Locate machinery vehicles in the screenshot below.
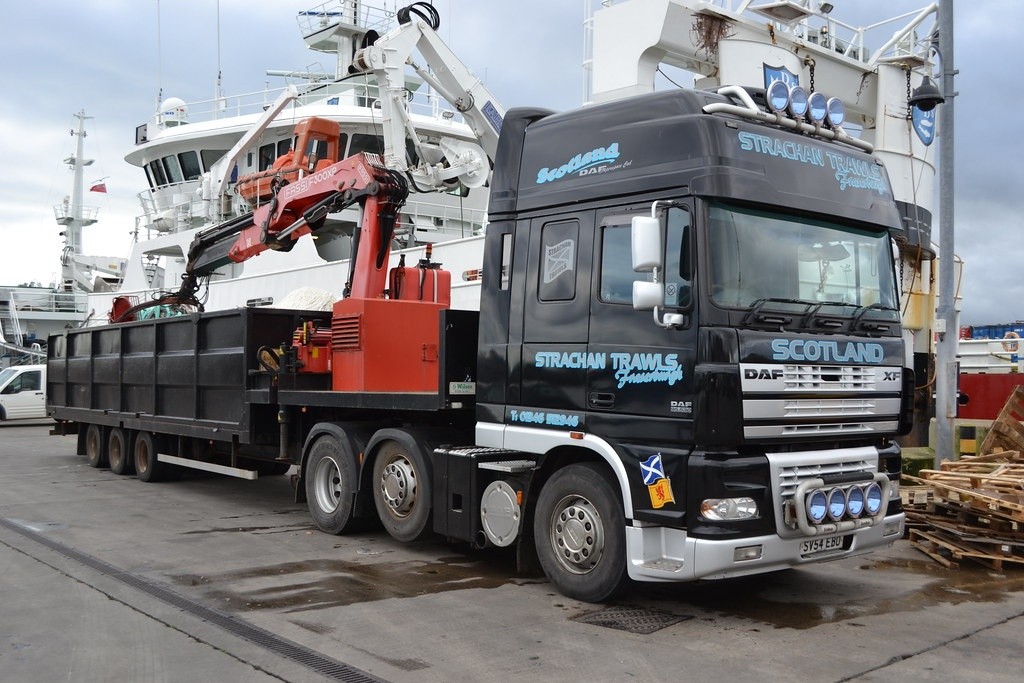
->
[113,114,455,395]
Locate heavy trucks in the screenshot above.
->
[44,78,918,604]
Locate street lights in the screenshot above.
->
[905,0,956,471]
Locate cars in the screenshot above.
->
[0,365,47,421]
